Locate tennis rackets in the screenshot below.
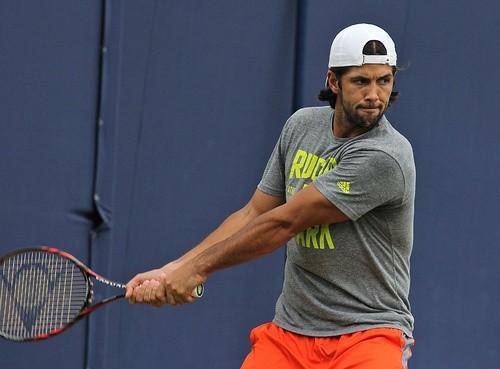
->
[0,245,203,343]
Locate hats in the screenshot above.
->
[325,24,398,86]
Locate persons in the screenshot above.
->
[125,23,417,368]
